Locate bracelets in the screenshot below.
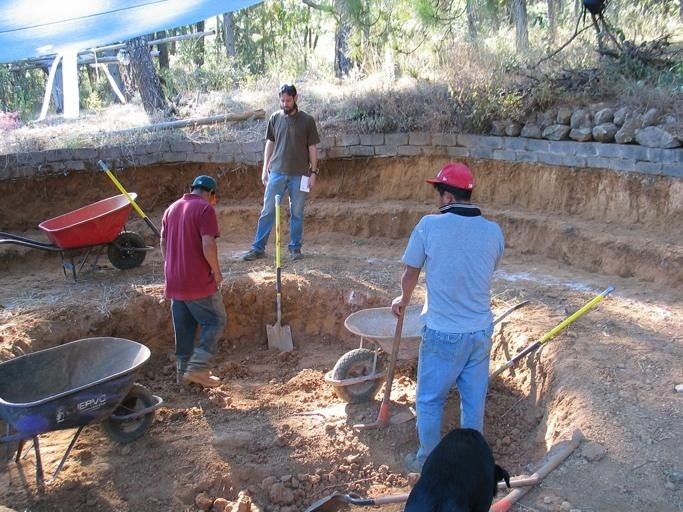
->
[311,169,319,175]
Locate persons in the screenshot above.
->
[391,162,505,473]
[159,175,227,388]
[243,83,320,264]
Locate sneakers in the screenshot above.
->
[404,453,419,473]
[183,371,221,387]
[243,249,266,260]
[289,249,301,261]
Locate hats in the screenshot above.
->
[425,163,475,189]
[192,175,216,195]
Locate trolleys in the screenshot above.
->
[323,287,531,405]
[0,192,155,285]
[1,337,163,490]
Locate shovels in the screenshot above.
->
[451,287,614,398]
[307,477,542,512]
[266,196,293,354]
